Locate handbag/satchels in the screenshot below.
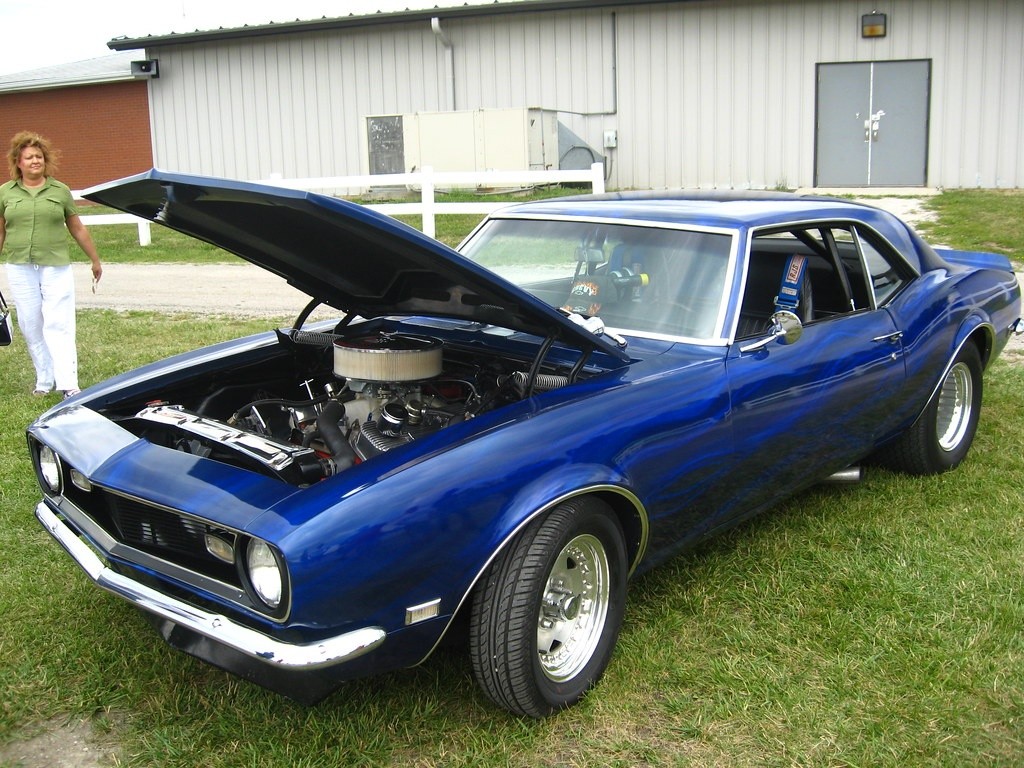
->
[0,291,13,346]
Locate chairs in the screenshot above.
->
[738,263,783,337]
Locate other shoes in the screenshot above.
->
[64,389,81,401]
[33,388,49,395]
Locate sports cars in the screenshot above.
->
[21,167,1022,722]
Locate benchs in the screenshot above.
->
[733,251,869,341]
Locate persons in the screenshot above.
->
[0,130,102,400]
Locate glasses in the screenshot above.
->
[93,276,97,294]
